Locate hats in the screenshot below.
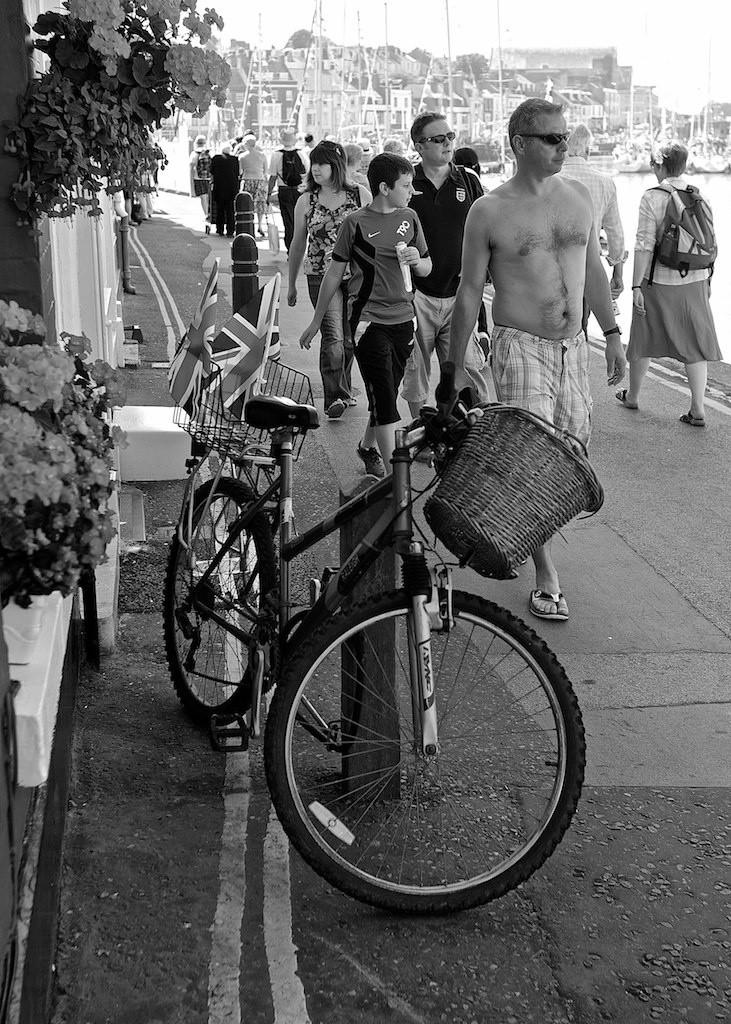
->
[356,138,373,154]
[279,129,297,146]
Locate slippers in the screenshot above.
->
[530,590,570,620]
[679,411,705,426]
[616,389,638,409]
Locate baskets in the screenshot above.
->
[172,357,315,461]
[423,406,603,581]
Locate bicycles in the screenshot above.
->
[158,347,609,915]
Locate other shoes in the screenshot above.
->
[347,396,357,406]
[328,398,344,418]
[258,229,265,237]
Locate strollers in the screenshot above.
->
[203,181,238,237]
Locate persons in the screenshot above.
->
[300,154,433,479]
[448,97,629,621]
[190,129,413,261]
[612,142,723,425]
[556,121,629,332]
[286,140,372,418]
[401,112,484,461]
[453,146,491,364]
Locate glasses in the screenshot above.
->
[419,132,456,143]
[319,141,342,157]
[650,161,664,168]
[512,131,570,145]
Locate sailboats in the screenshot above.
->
[235,1,731,176]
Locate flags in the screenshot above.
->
[165,256,219,421]
[211,273,283,427]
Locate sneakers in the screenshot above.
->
[356,437,385,478]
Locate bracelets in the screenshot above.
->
[603,324,623,337]
[632,286,642,290]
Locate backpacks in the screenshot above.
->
[276,149,303,186]
[197,150,212,178]
[646,183,717,270]
